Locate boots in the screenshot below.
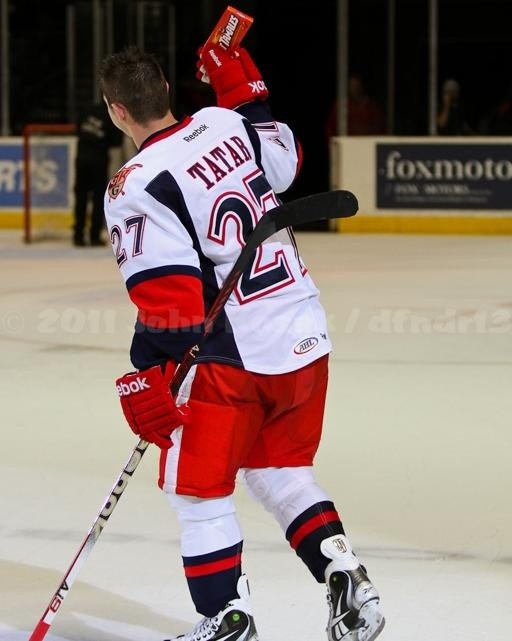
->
[164,573,257,641]
[321,534,381,640]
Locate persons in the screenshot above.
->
[93,39,393,641]
[432,74,469,136]
[70,99,125,251]
[324,72,384,138]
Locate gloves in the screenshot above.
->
[115,359,194,448]
[194,47,267,110]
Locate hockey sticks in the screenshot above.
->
[33,190,358,641]
